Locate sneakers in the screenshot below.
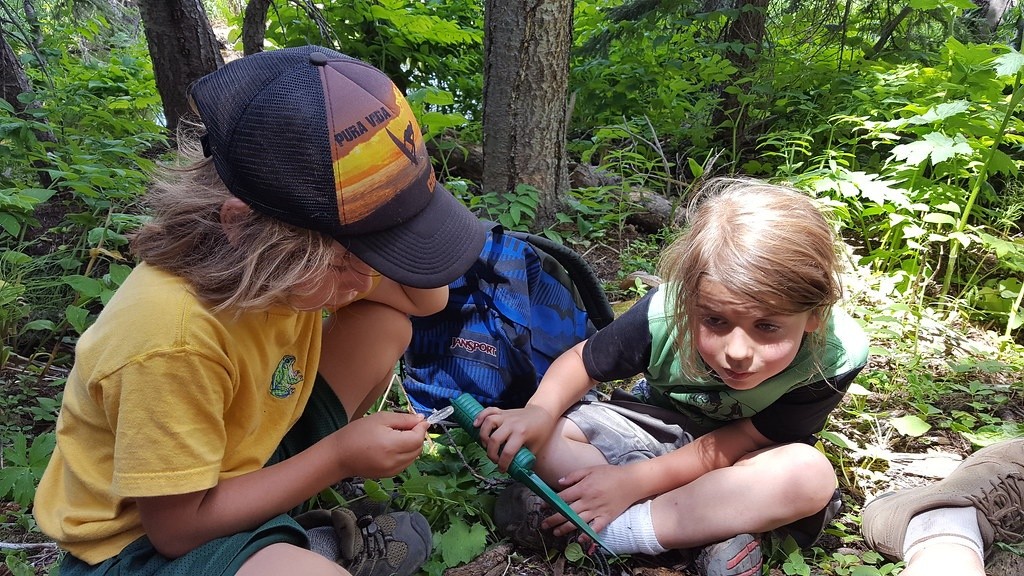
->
[860,437,1024,564]
[293,508,432,576]
[312,478,407,519]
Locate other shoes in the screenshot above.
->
[689,533,764,576]
[494,482,596,557]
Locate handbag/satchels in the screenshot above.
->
[399,219,617,420]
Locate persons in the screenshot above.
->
[33,45,486,576]
[473,176,869,576]
[862,437,1024,576]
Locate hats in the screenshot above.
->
[185,44,487,289]
